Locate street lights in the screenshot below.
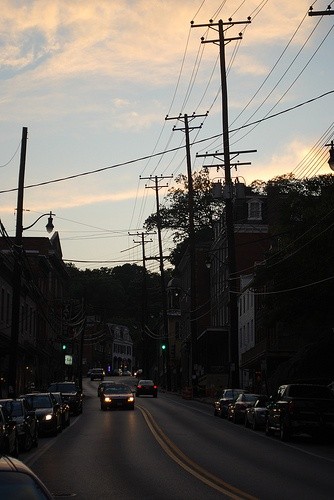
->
[8,211,55,397]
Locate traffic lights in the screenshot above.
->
[62,345,66,350]
[161,345,166,349]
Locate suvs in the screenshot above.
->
[265,383,334,440]
[91,368,104,381]
[48,381,84,415]
[0,396,42,457]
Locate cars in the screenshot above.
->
[214,388,270,430]
[97,380,136,410]
[0,454,56,500]
[23,391,72,437]
[135,380,158,397]
